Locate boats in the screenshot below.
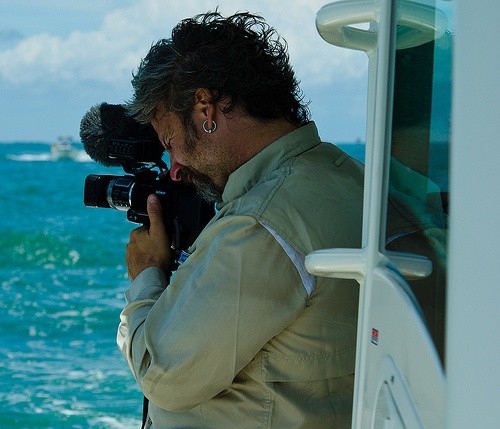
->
[51,135,76,160]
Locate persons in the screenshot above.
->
[116,4,364,429]
